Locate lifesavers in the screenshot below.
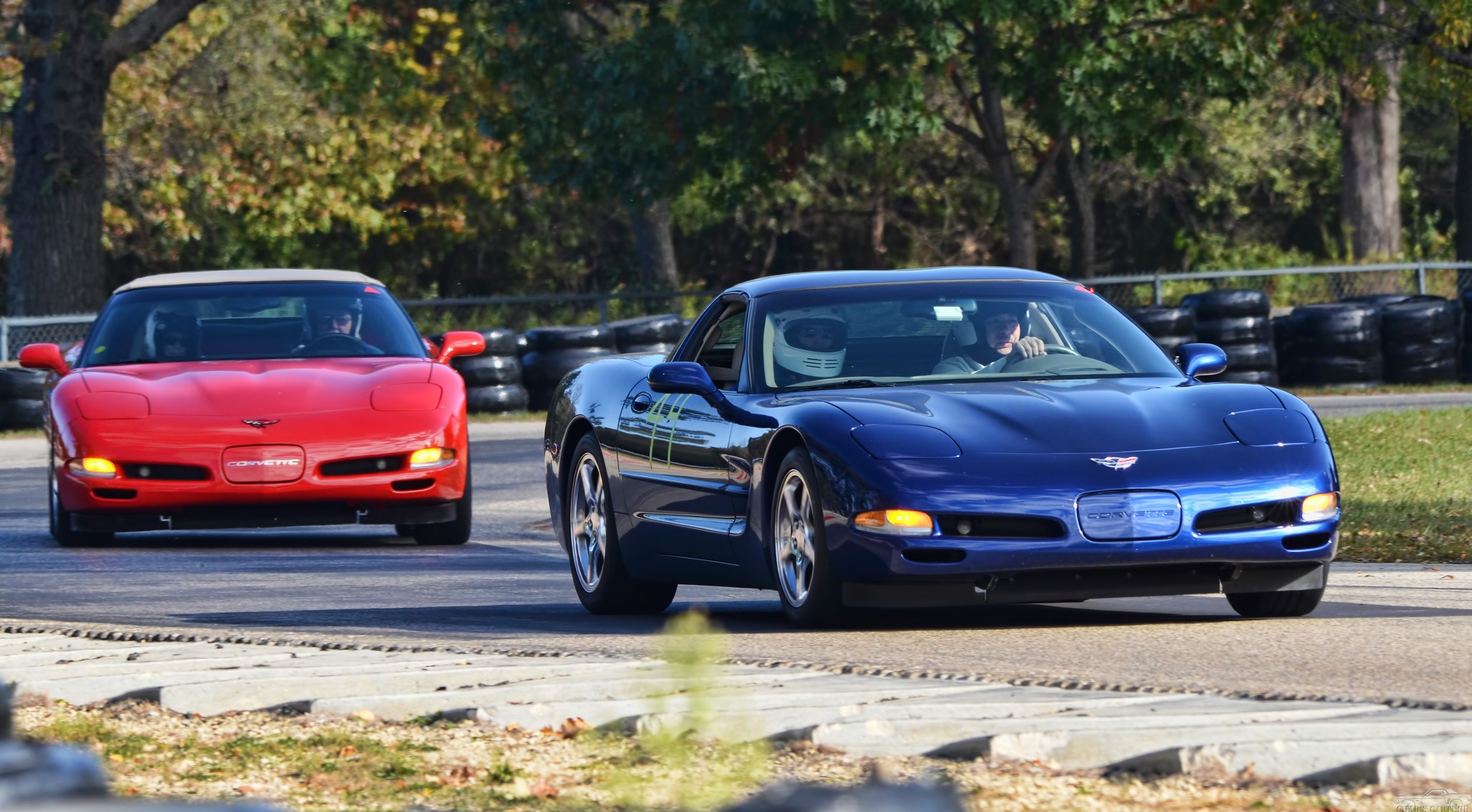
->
[524,312,685,409]
[2,365,50,428]
[1289,293,1461,387]
[1115,287,1281,389]
[428,326,530,413]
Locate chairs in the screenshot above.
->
[942,312,985,364]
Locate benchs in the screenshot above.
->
[701,335,945,391]
[198,317,306,357]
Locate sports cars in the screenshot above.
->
[540,268,1343,631]
[15,269,485,548]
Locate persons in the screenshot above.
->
[143,298,205,359]
[768,304,867,387]
[292,294,363,353]
[930,303,1047,376]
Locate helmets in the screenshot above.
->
[952,302,1031,347]
[141,304,203,358]
[302,296,362,341]
[772,306,849,378]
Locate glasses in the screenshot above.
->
[317,316,351,326]
[162,332,195,349]
[792,326,835,340]
